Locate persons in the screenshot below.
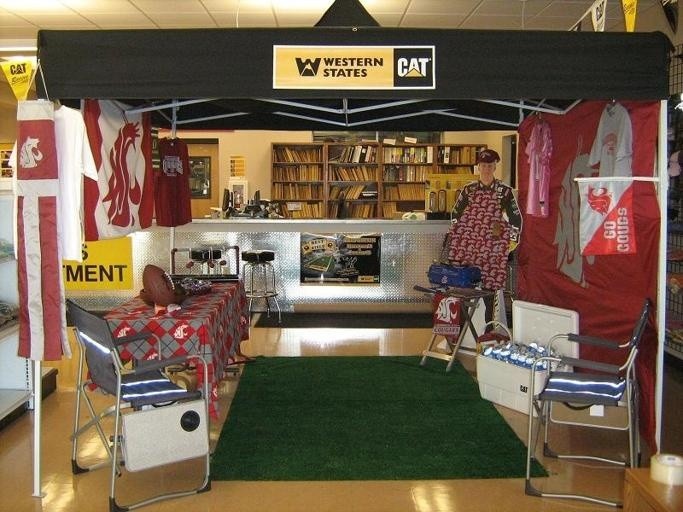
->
[604,135,618,155]
[450,150,524,342]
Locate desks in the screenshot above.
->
[623,466,683,512]
[87,280,253,420]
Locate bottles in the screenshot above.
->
[481,336,555,371]
[667,231,683,354]
[233,190,243,208]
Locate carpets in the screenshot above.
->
[208,355,549,481]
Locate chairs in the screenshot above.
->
[525,298,651,507]
[64,300,211,511]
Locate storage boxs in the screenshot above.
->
[475,300,580,417]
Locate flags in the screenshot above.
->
[80,98,155,242]
[578,178,638,256]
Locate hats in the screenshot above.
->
[474,149,501,166]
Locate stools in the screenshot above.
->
[413,285,497,372]
[241,250,281,328]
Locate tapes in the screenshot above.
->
[651,453,683,486]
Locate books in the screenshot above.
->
[273,137,486,218]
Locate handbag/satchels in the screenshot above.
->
[428,258,482,289]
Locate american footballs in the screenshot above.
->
[141,265,174,306]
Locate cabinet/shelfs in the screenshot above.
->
[269,142,488,219]
